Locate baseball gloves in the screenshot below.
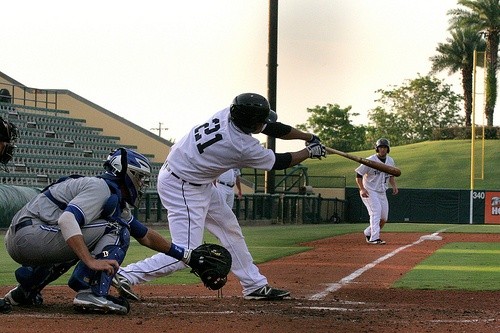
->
[189,243,232,290]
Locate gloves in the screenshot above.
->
[306,143,327,160]
[306,134,321,146]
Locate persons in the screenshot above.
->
[354,139,398,243]
[0,148,232,311]
[111,93,327,301]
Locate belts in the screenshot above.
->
[165,165,202,187]
[15,220,33,231]
[220,181,234,188]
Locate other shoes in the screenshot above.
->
[366,236,385,244]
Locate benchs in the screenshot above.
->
[0,99,165,221]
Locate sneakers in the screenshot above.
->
[4,287,43,306]
[244,285,290,300]
[112,273,139,301]
[72,292,127,314]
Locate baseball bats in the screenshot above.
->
[325,146,401,178]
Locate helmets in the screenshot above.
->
[103,147,152,210]
[230,93,277,132]
[375,138,390,154]
